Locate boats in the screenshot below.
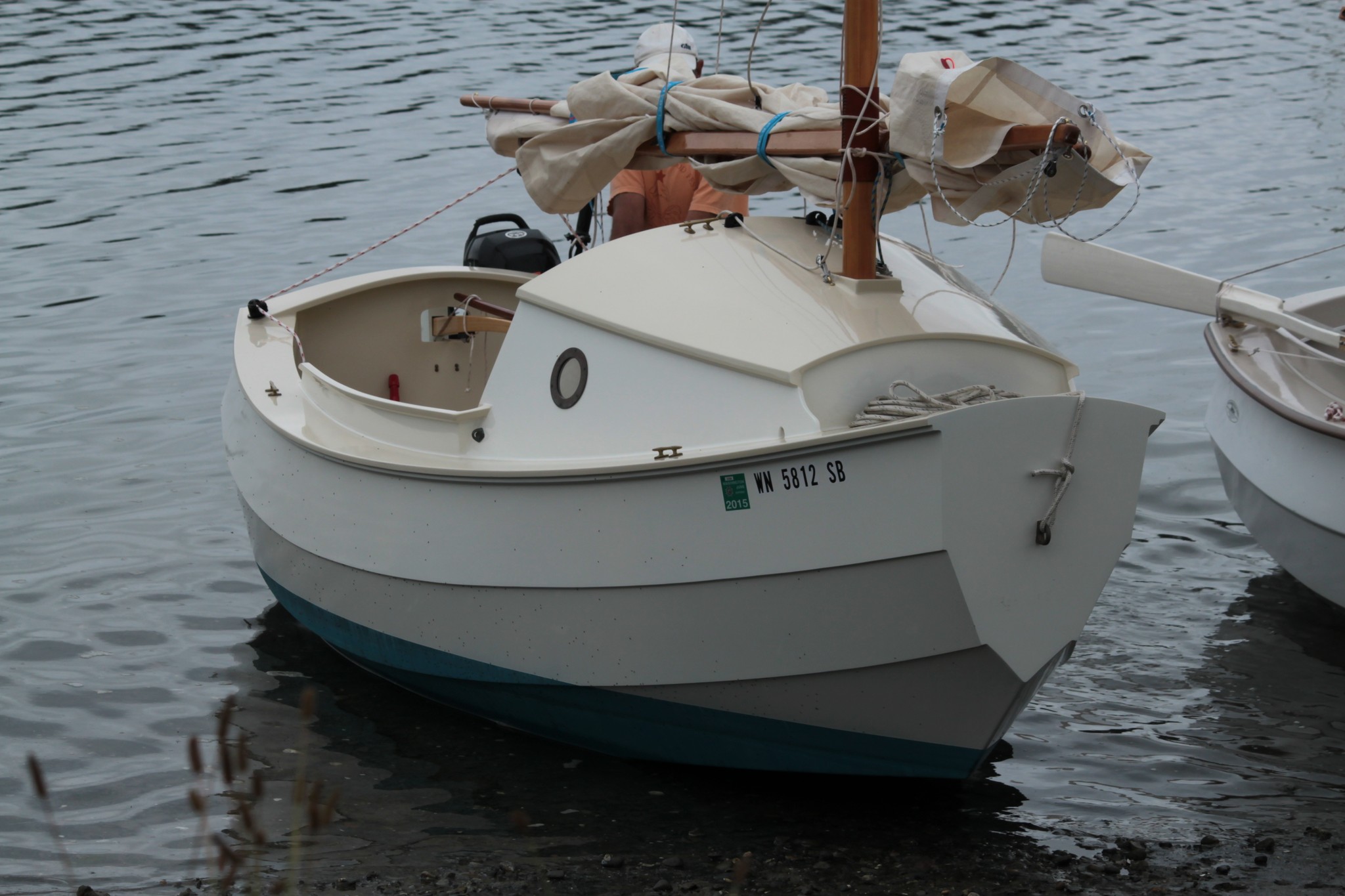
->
[1203,285,1345,610]
[227,1,1169,784]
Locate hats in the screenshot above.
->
[634,22,699,73]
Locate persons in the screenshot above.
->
[607,21,749,241]
